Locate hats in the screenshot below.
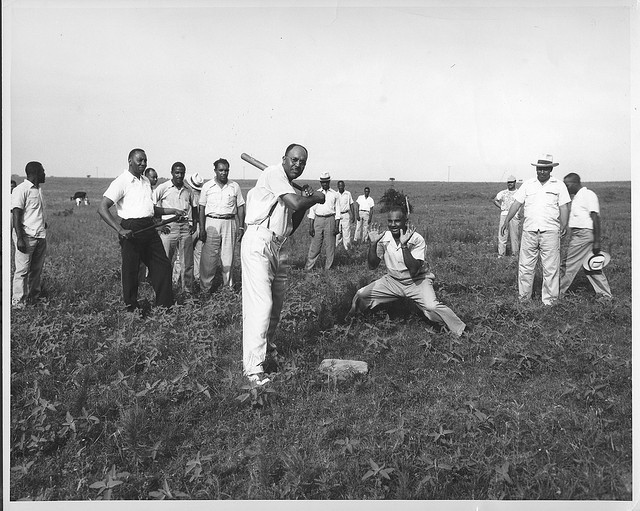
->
[319,171,331,182]
[185,172,204,191]
[507,175,516,183]
[529,154,559,168]
[582,250,611,272]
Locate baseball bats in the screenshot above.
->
[121,214,181,240]
[241,153,304,191]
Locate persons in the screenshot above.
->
[84,195,89,206]
[239,143,326,395]
[336,181,355,251]
[75,194,83,207]
[492,176,521,258]
[10,181,17,228]
[500,152,572,306]
[354,187,375,243]
[151,162,198,292]
[144,168,158,190]
[97,148,172,311]
[344,187,467,340]
[560,173,612,299]
[12,162,48,311]
[303,171,342,269]
[198,158,246,298]
[172,170,203,286]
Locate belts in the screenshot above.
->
[206,214,231,220]
[342,211,348,213]
[315,213,334,217]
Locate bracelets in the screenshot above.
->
[237,227,245,231]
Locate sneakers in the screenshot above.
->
[247,373,270,387]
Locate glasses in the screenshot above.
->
[287,155,306,167]
